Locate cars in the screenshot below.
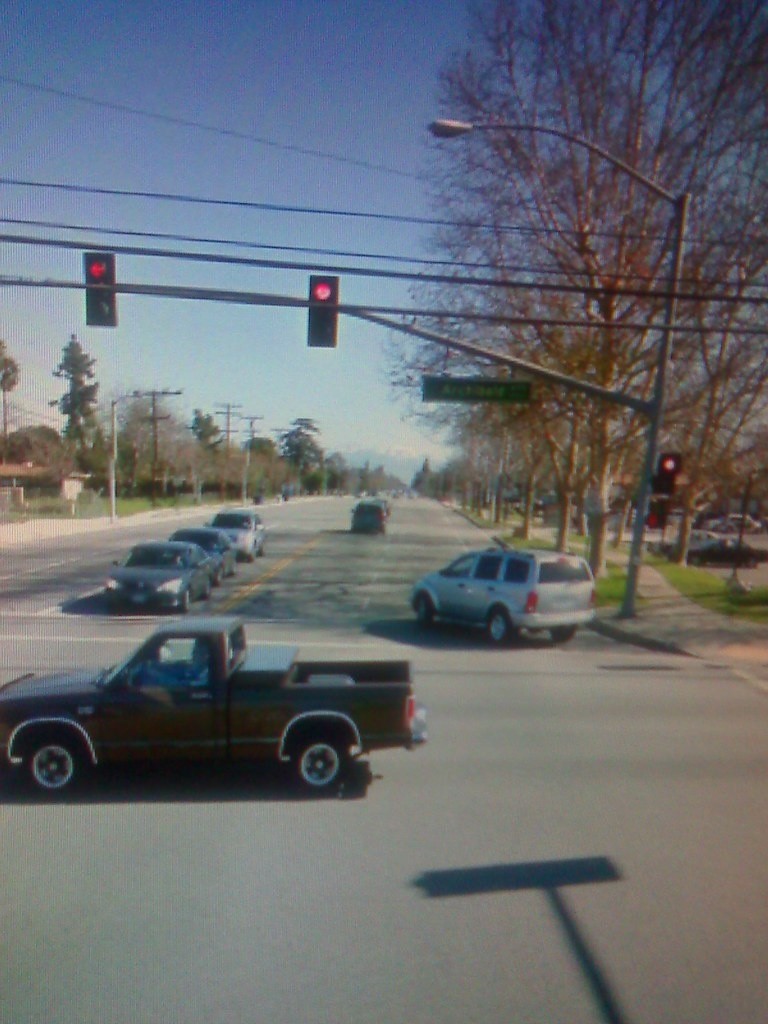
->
[350,500,390,535]
[676,528,733,554]
[104,539,224,613]
[205,509,265,561]
[712,514,762,535]
[168,527,238,578]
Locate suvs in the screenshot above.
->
[410,546,600,644]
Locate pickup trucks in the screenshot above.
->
[0,615,429,791]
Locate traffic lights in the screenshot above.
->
[657,452,679,501]
[81,250,118,329]
[307,275,341,349]
[647,501,669,530]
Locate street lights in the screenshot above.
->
[427,117,695,619]
[110,394,143,522]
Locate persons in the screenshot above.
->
[164,551,184,568]
[162,638,210,687]
